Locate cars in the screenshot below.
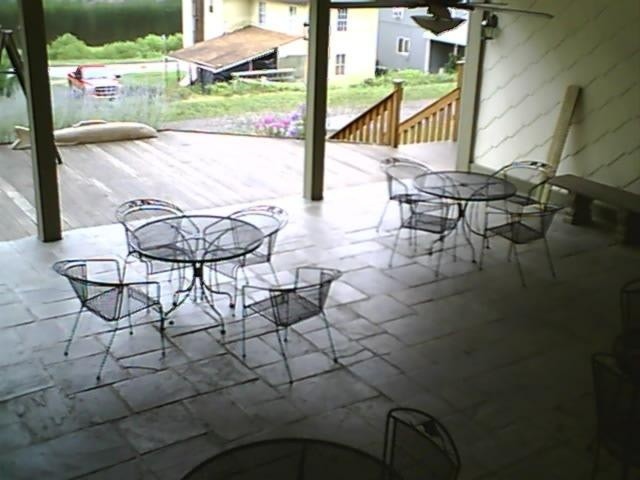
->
[67,63,122,102]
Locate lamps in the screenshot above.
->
[412,15,468,35]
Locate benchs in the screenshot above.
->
[548,174,640,239]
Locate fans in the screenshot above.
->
[325,0,556,19]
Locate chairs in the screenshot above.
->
[477,183,571,287]
[115,198,200,314]
[203,204,288,318]
[388,187,463,277]
[241,266,342,386]
[487,161,557,263]
[611,279,640,370]
[375,156,447,232]
[52,259,165,381]
[591,351,640,479]
[384,406,461,480]
